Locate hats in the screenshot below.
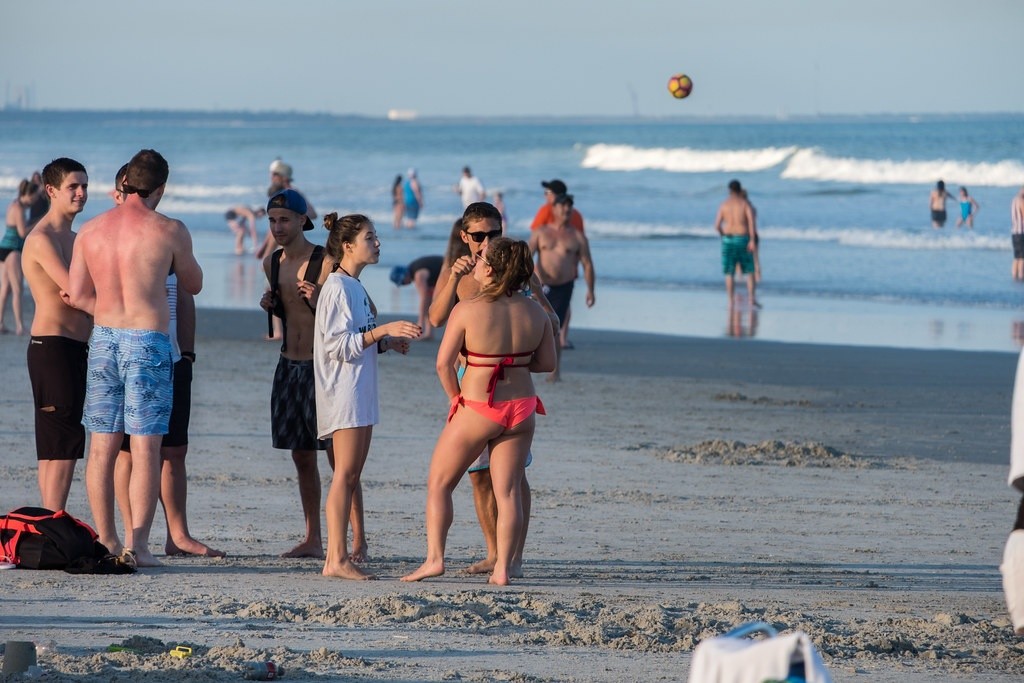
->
[542,180,566,196]
[267,189,314,231]
[390,266,409,288]
[270,160,294,182]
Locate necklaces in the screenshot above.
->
[334,263,360,282]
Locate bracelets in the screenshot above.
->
[181,351,196,362]
[370,330,376,343]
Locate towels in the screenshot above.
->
[685,630,837,683]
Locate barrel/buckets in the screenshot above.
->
[2,640,37,682]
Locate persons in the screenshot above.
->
[1011,186,1024,280]
[529,179,583,349]
[999,346,1024,634]
[313,213,424,580]
[529,194,595,329]
[929,181,979,227]
[715,178,763,310]
[260,190,371,562]
[114,164,228,559]
[21,157,96,511]
[429,201,561,577]
[390,218,473,341]
[224,158,317,340]
[70,147,203,567]
[392,168,423,226]
[453,166,486,207]
[399,236,557,585]
[495,192,507,228]
[0,171,50,336]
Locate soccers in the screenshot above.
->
[668,74,693,100]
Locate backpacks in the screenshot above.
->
[0,506,138,574]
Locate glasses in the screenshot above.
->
[464,228,501,242]
[122,178,166,198]
[475,250,497,272]
